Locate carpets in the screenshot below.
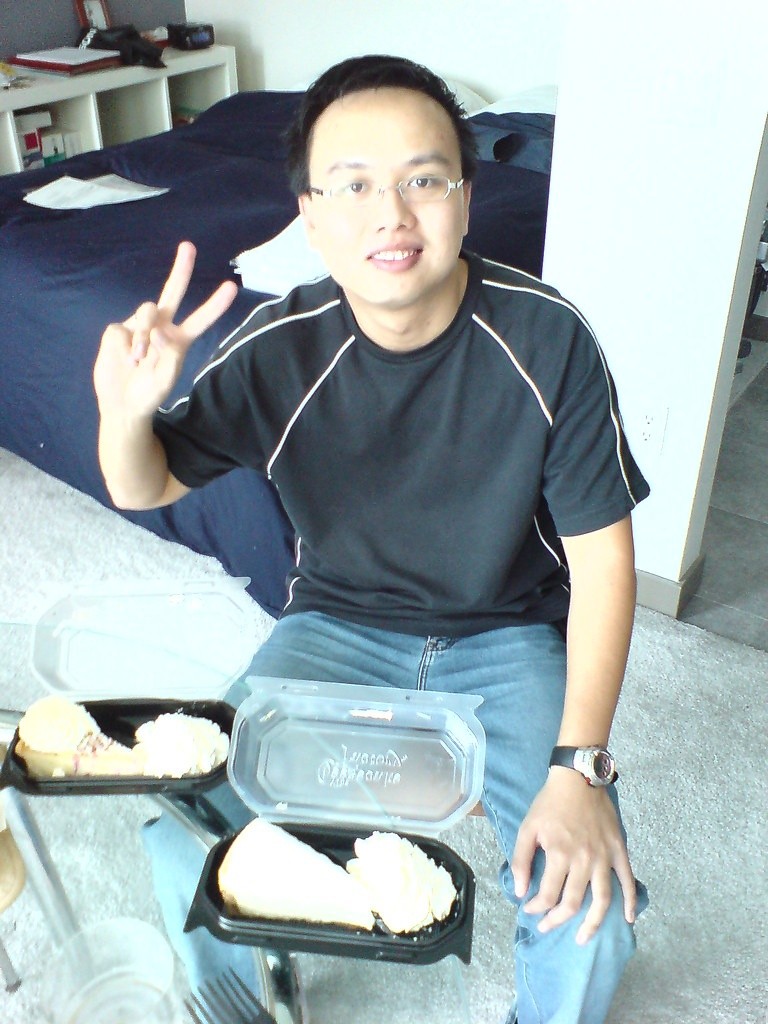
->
[0,449,768,1024]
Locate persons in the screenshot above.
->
[93,57,650,1024]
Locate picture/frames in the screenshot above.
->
[71,0,111,32]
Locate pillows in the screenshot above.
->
[444,79,555,172]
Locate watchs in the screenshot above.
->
[548,745,620,789]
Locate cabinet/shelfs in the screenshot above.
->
[0,40,239,175]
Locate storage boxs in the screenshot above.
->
[15,110,80,173]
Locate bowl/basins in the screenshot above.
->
[2,692,238,793]
[185,675,485,969]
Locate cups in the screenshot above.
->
[33,913,185,1024]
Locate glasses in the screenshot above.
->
[309,173,464,208]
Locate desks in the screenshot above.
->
[0,619,471,1024]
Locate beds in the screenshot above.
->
[0,91,555,621]
